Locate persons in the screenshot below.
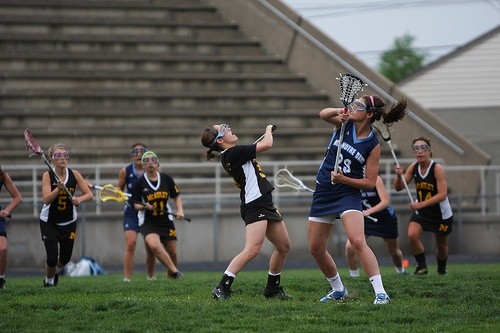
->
[394,136,454,275]
[307,95,409,303]
[130,151,186,279]
[0,169,22,290]
[39,144,94,287]
[344,166,406,277]
[200,124,293,300]
[113,142,156,282]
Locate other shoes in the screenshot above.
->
[412,266,428,278]
[0,278,6,290]
[374,293,391,306]
[167,269,183,281]
[396,270,410,277]
[320,287,349,302]
[211,286,233,300]
[437,265,448,276]
[263,286,293,301]
[44,273,59,287]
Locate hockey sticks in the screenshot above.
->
[371,115,419,215]
[330,73,365,185]
[100,184,191,222]
[274,169,378,222]
[24,128,79,207]
[89,184,132,197]
[253,125,277,144]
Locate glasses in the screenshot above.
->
[52,151,70,160]
[412,143,430,152]
[143,157,155,162]
[214,122,229,141]
[349,99,368,111]
[131,149,146,155]
